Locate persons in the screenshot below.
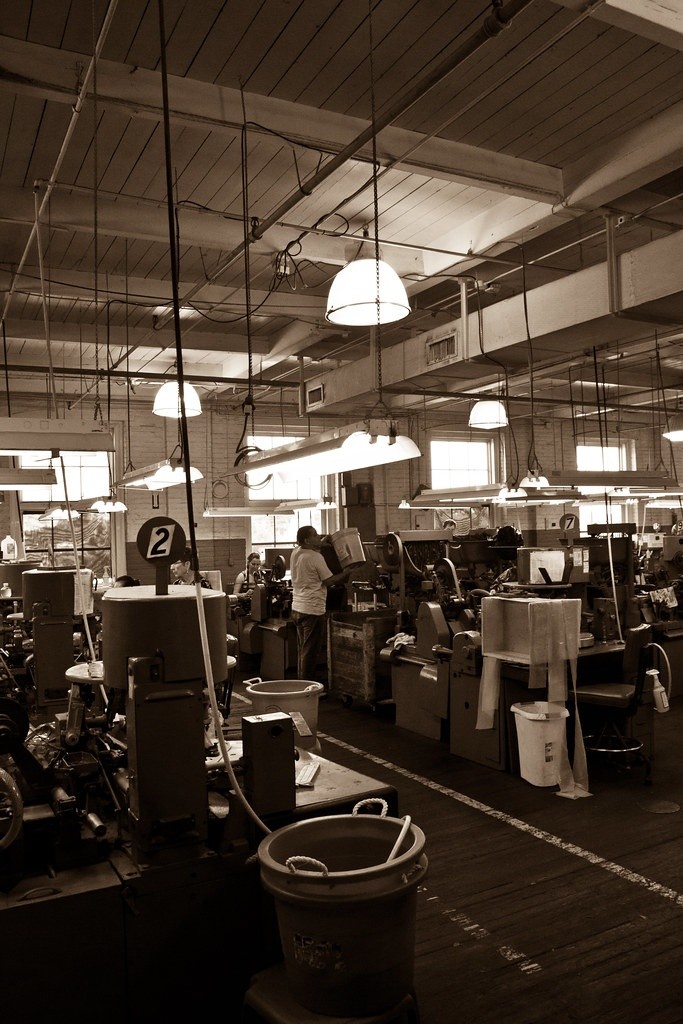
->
[233,552,260,611]
[290,525,360,697]
[170,547,212,589]
[114,575,140,587]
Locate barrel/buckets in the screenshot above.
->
[463,541,495,562]
[243,677,324,750]
[584,720,644,783]
[330,528,366,569]
[257,798,429,1017]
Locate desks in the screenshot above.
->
[502,582,572,600]
[65,661,104,730]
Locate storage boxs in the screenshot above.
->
[521,529,575,547]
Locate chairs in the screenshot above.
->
[568,623,654,788]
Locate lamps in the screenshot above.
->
[325,223,412,326]
[109,236,204,490]
[88,448,128,514]
[35,458,81,521]
[152,358,202,418]
[397,241,683,510]
[202,404,337,518]
[220,0,421,490]
[468,390,509,431]
[661,391,683,441]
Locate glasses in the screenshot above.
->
[250,561,260,566]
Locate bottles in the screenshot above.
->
[1,535,18,564]
[1,583,12,598]
[653,675,670,713]
[74,566,94,615]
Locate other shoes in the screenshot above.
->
[318,691,327,696]
